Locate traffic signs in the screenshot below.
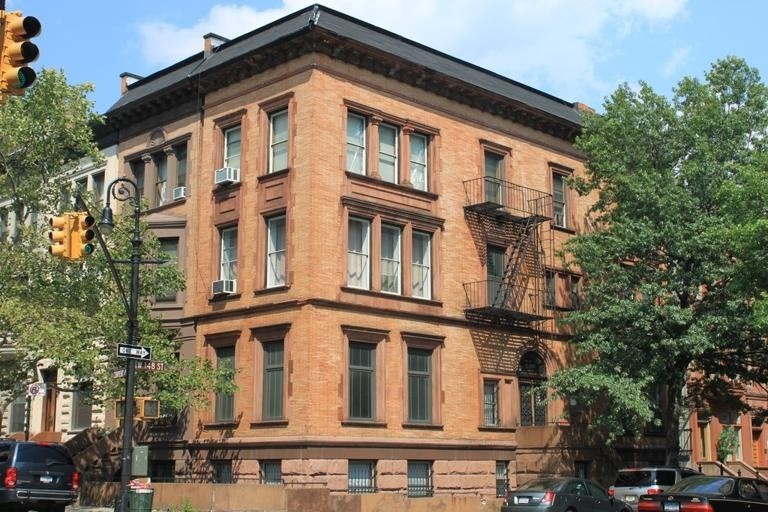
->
[116,343,152,361]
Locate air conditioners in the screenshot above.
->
[213,167,241,185]
[211,279,236,297]
[172,184,187,201]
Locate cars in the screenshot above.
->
[501,466,768,512]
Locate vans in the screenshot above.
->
[0,438,81,512]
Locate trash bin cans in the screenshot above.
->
[127,488,154,512]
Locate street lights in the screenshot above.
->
[98,175,143,512]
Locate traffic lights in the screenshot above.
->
[49,216,65,257]
[81,215,96,256]
[115,398,160,421]
[5,15,41,89]
